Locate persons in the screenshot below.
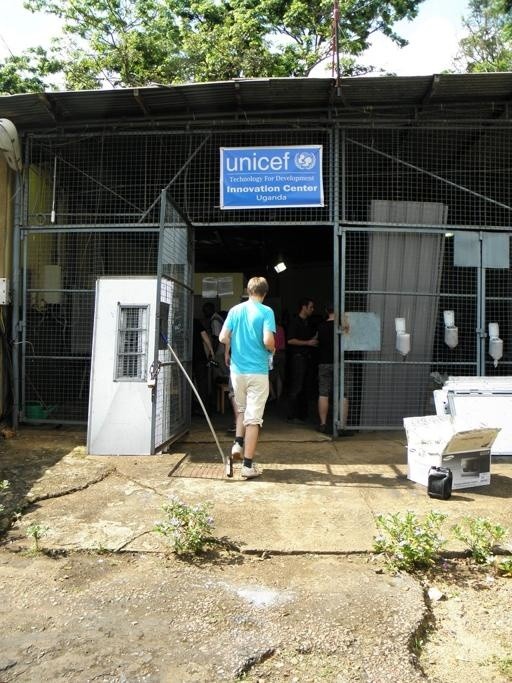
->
[193,297,354,437]
[218,277,277,478]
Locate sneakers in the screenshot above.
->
[287,418,354,436]
[241,463,259,477]
[231,442,244,460]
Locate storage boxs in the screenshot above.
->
[402,415,502,490]
